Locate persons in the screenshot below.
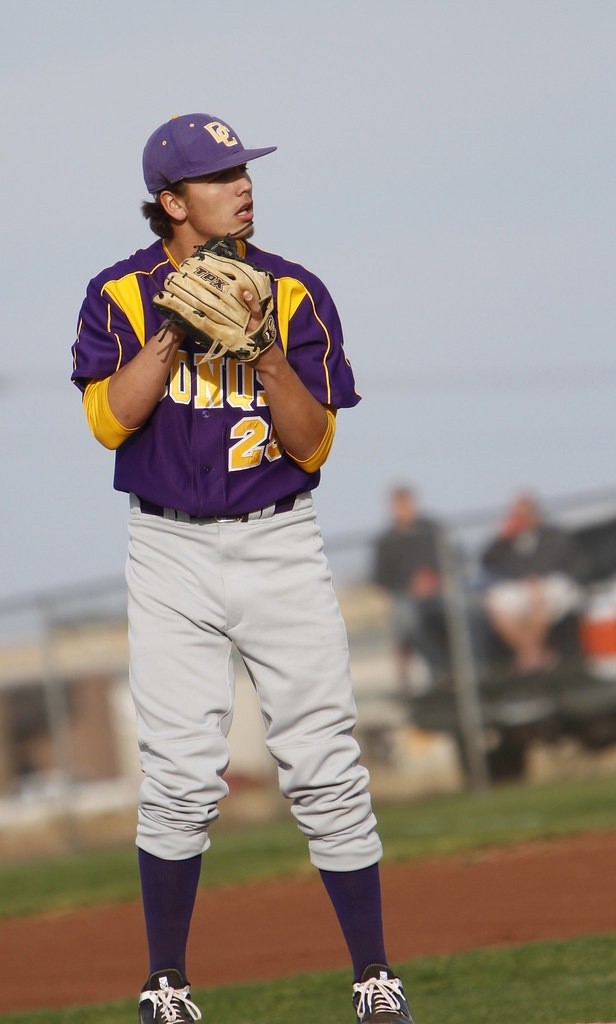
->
[72,114,412,1024]
[375,489,467,696]
[477,500,592,688]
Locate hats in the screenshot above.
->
[143,113,277,193]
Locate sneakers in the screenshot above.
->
[138,969,202,1024]
[352,964,415,1024]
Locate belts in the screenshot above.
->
[140,499,294,523]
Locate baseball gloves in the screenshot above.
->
[151,235,278,362]
